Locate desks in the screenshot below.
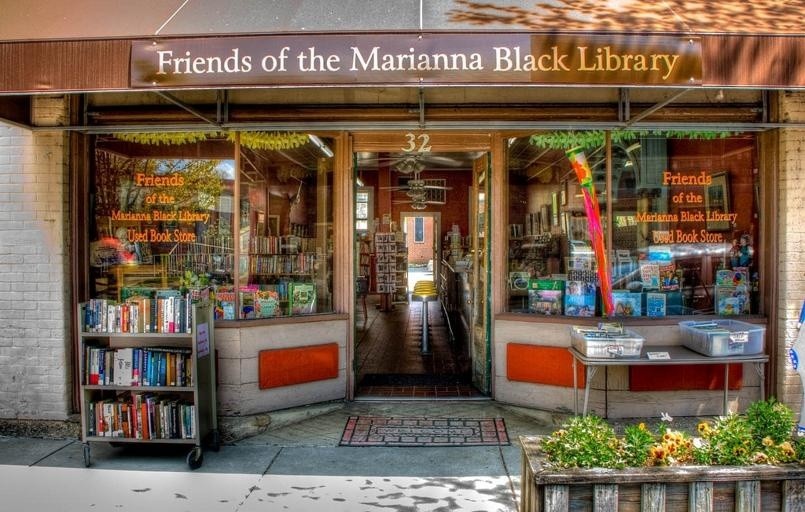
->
[109,265,167,303]
[568,346,770,418]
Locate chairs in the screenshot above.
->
[355,274,370,319]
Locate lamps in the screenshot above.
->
[357,161,364,188]
[411,203,427,210]
[309,134,334,158]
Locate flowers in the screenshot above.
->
[538,395,805,470]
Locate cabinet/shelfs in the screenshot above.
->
[75,332,202,445]
[208,253,316,302]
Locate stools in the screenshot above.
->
[412,281,439,356]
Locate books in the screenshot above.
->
[214,234,319,323]
[82,234,214,440]
[508,238,755,317]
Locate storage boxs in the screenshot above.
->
[678,319,767,356]
[568,323,645,358]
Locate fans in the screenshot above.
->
[360,153,464,205]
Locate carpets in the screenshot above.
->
[340,415,511,447]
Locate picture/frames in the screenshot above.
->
[563,250,633,277]
[703,171,733,232]
[217,194,250,235]
[509,178,566,241]
